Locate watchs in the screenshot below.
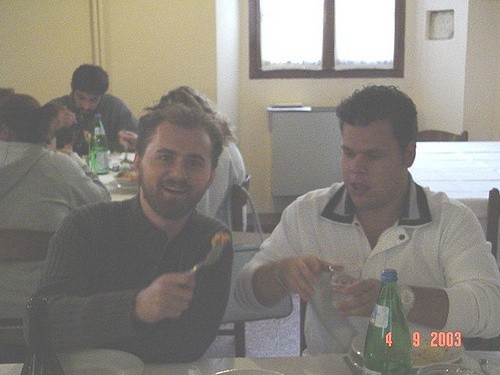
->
[395,284,416,319]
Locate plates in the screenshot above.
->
[216,368,280,375]
[347,324,500,375]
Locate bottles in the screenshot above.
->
[87,114,109,175]
[362,268,412,375]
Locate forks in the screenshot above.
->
[184,231,223,276]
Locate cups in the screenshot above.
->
[328,263,363,314]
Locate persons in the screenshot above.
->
[0,94,110,361]
[37,62,246,217]
[19,97,234,375]
[233,81,500,355]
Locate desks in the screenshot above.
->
[98,152,136,201]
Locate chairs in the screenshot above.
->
[217,174,251,357]
[0,227,56,343]
